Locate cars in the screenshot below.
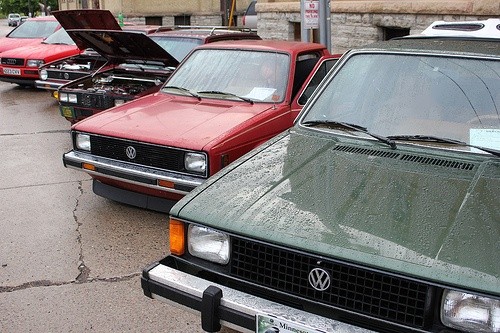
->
[8,14,28,27]
[57,25,262,128]
[141,19,500,333]
[0,22,135,92]
[0,16,63,52]
[35,10,181,98]
[63,40,345,214]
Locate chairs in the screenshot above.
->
[374,73,441,136]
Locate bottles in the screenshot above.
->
[118,12,124,27]
